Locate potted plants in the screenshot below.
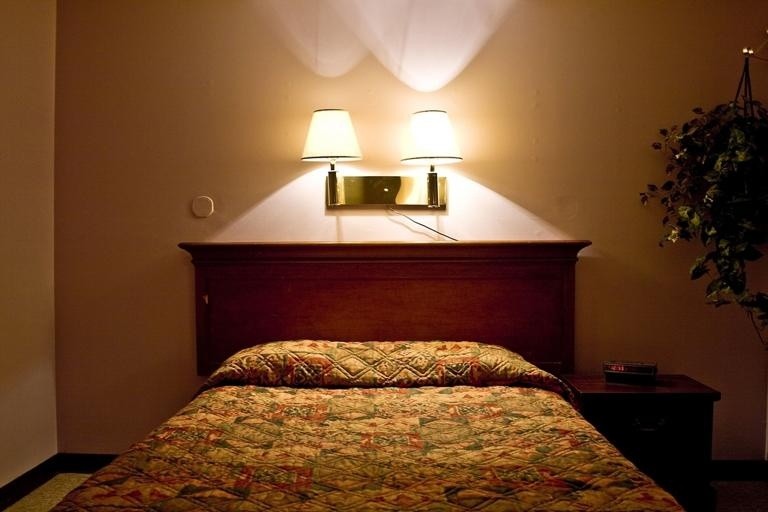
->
[639,45,768,352]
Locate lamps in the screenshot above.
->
[301,109,361,207]
[401,110,463,208]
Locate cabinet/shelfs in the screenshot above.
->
[563,374,722,479]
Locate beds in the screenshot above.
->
[51,240,687,512]
